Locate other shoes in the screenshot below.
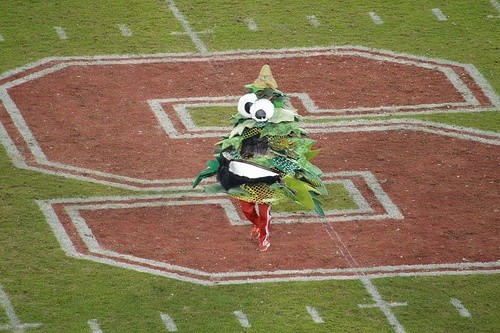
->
[256,237,270,252]
[250,225,260,240]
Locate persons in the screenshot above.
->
[193,65,329,253]
